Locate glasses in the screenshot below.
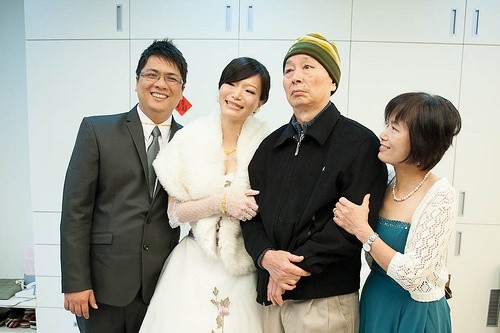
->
[140,71,184,85]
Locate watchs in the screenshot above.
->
[363,233,379,252]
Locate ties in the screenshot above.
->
[146,125,162,205]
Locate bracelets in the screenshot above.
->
[221,192,227,213]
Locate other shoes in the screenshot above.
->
[0,309,36,329]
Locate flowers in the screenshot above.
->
[209,287,232,333]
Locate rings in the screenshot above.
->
[333,208,337,212]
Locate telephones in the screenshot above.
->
[14,282,36,300]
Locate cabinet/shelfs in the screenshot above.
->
[23,0,500,333]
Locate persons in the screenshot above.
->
[137,57,284,333]
[60,37,188,333]
[242,32,389,333]
[333,92,462,333]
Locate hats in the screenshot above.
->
[283,32,341,96]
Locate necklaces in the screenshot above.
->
[224,148,237,154]
[392,170,432,202]
